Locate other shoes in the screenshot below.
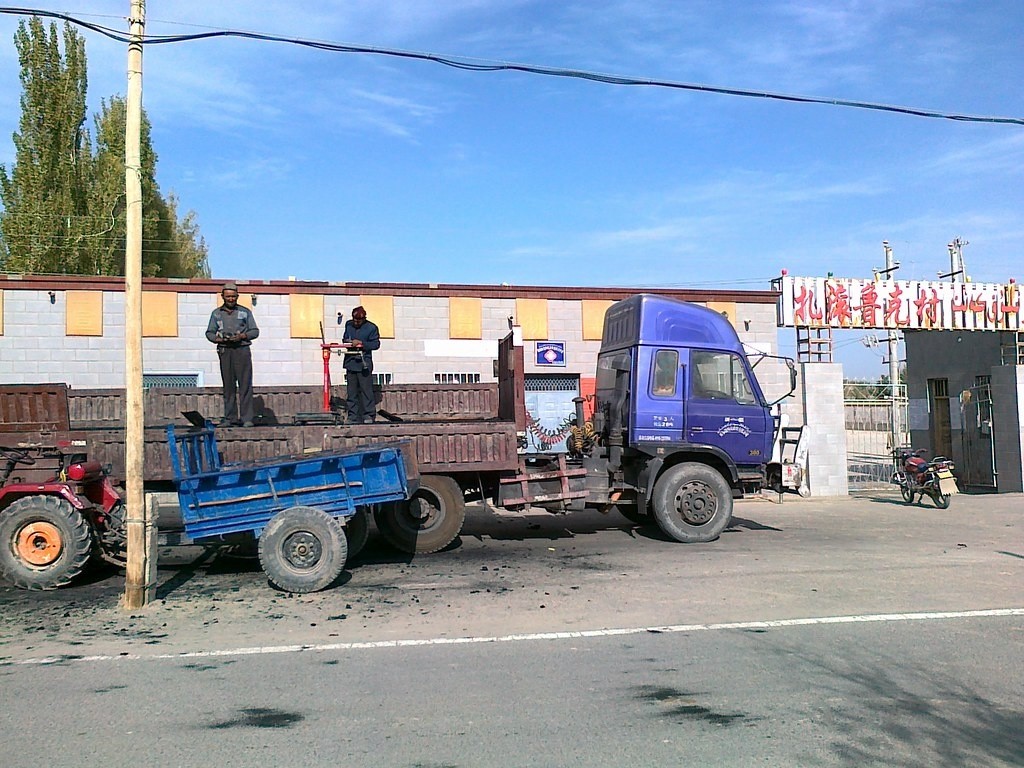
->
[344,418,357,424]
[364,418,373,424]
[243,421,254,427]
[217,419,240,427]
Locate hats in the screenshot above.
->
[223,283,237,292]
[352,306,366,318]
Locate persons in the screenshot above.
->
[341,306,381,425]
[205,283,259,428]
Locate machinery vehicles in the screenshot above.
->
[0,410,420,593]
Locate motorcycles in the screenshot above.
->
[889,446,960,508]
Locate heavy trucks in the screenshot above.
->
[0,295,799,557]
